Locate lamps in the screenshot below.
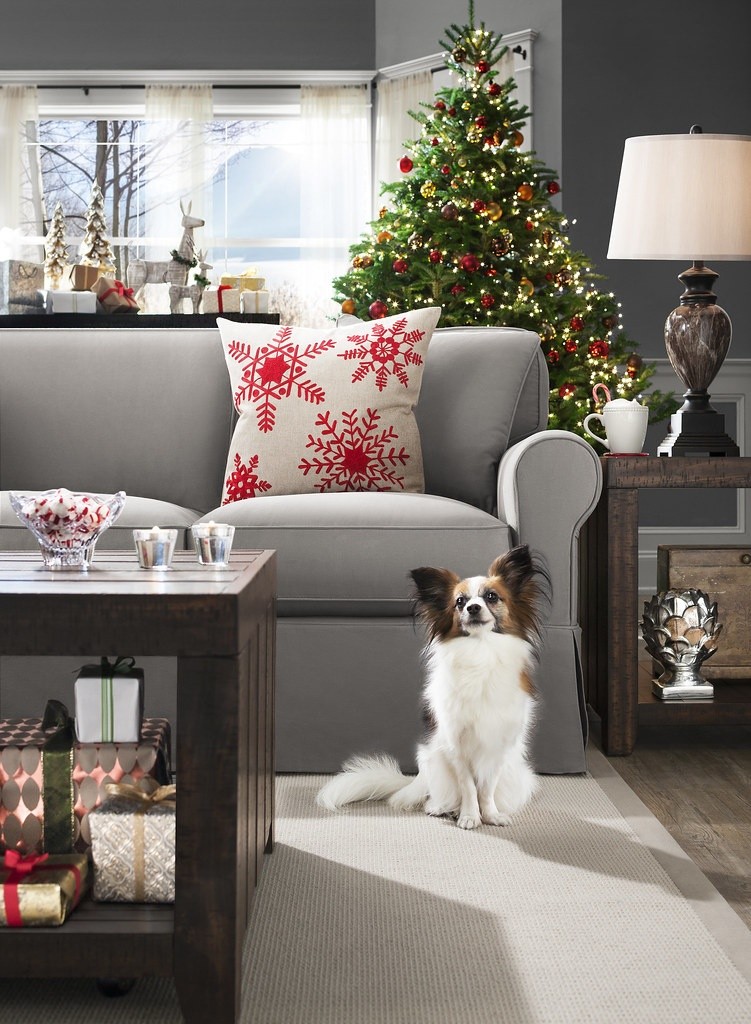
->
[607,122,751,456]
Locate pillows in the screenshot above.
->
[216,306,441,508]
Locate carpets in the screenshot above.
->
[0,740,751,1024]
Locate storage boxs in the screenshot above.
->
[89,782,176,903]
[73,656,145,743]
[202,286,240,313]
[222,268,266,293]
[0,851,90,927]
[657,545,751,680]
[239,288,269,313]
[0,718,171,856]
[91,277,141,313]
[46,289,96,313]
[63,264,98,291]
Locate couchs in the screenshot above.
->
[0,301,603,776]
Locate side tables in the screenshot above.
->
[578,457,751,755]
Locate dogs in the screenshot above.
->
[316,543,553,830]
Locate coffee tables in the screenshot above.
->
[0,548,277,1024]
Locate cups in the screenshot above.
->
[134,530,177,568]
[584,398,649,452]
[191,526,236,565]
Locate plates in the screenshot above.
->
[8,489,126,565]
[604,453,649,457]
